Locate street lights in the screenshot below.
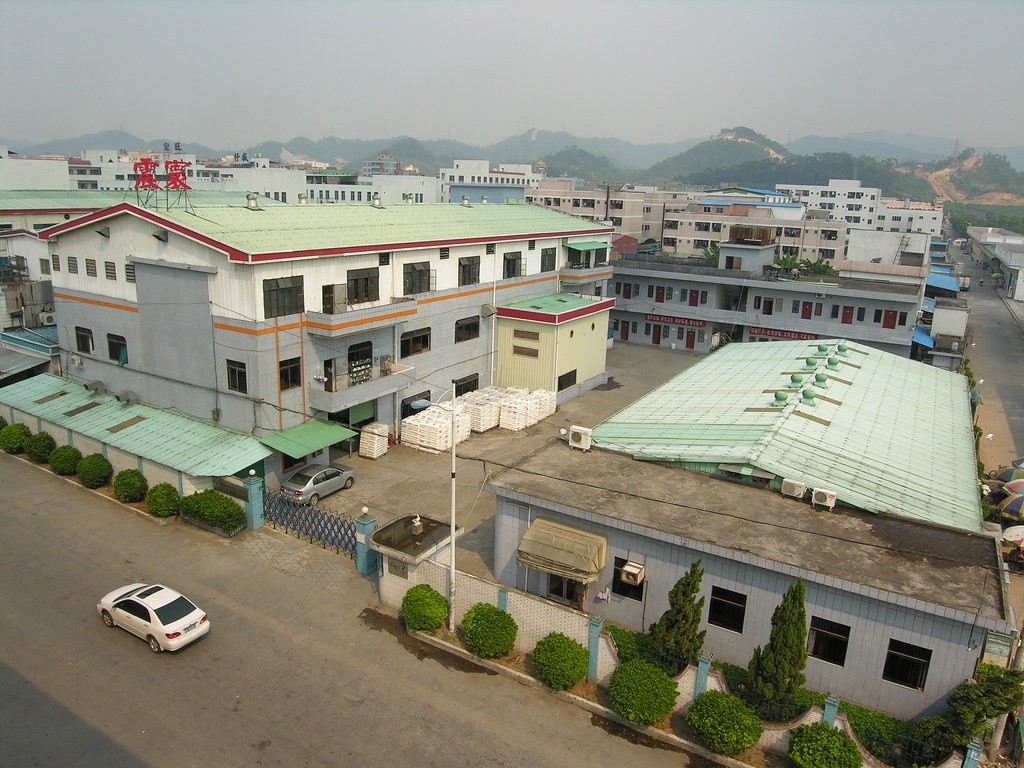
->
[410,379,455,635]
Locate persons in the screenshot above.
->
[570,581,588,611]
[1009,547,1024,570]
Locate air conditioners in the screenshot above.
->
[569,425,592,449]
[816,294,826,299]
[621,561,646,586]
[781,478,806,498]
[811,487,837,507]
[70,356,80,367]
[43,312,56,326]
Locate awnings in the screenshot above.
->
[563,242,613,252]
[912,326,933,349]
[516,517,607,583]
[260,419,359,460]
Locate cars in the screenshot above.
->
[97,582,211,654]
[279,463,356,508]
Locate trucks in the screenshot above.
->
[959,275,970,291]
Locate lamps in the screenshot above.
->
[249,469,256,476]
[362,507,368,514]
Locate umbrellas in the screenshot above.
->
[992,272,1003,284]
[996,456,1024,547]
